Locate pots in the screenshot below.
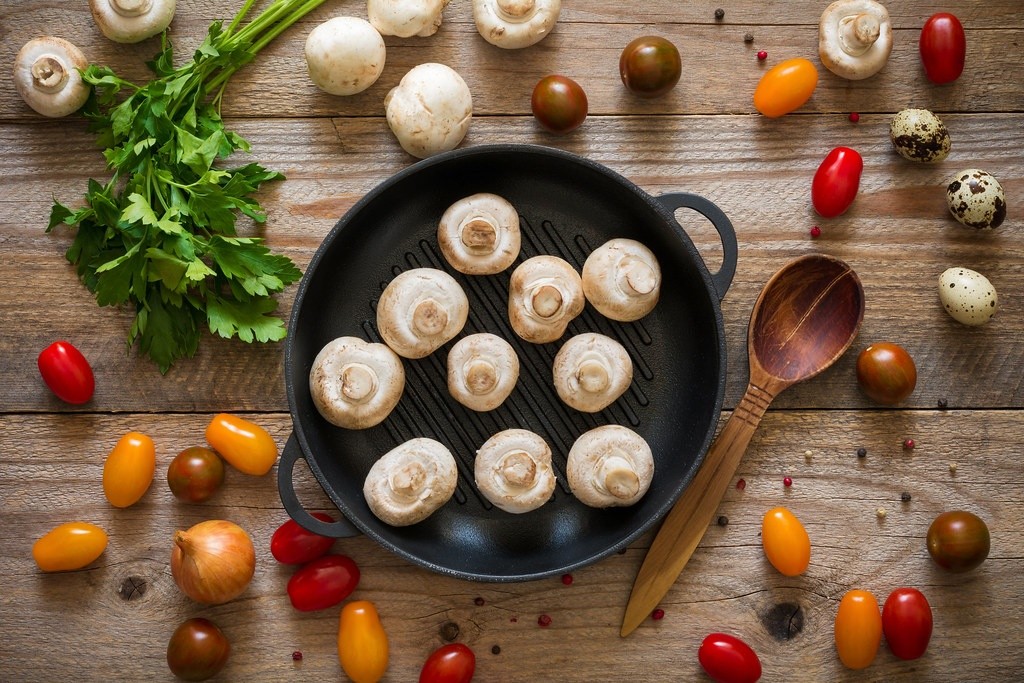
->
[269,140,740,589]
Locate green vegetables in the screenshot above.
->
[42,0,327,375]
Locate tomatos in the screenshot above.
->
[531,13,968,220]
[32,340,476,683]
[696,340,991,683]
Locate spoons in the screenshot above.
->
[619,252,866,641]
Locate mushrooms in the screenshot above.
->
[818,0,894,81]
[14,1,559,159]
[307,191,663,531]
